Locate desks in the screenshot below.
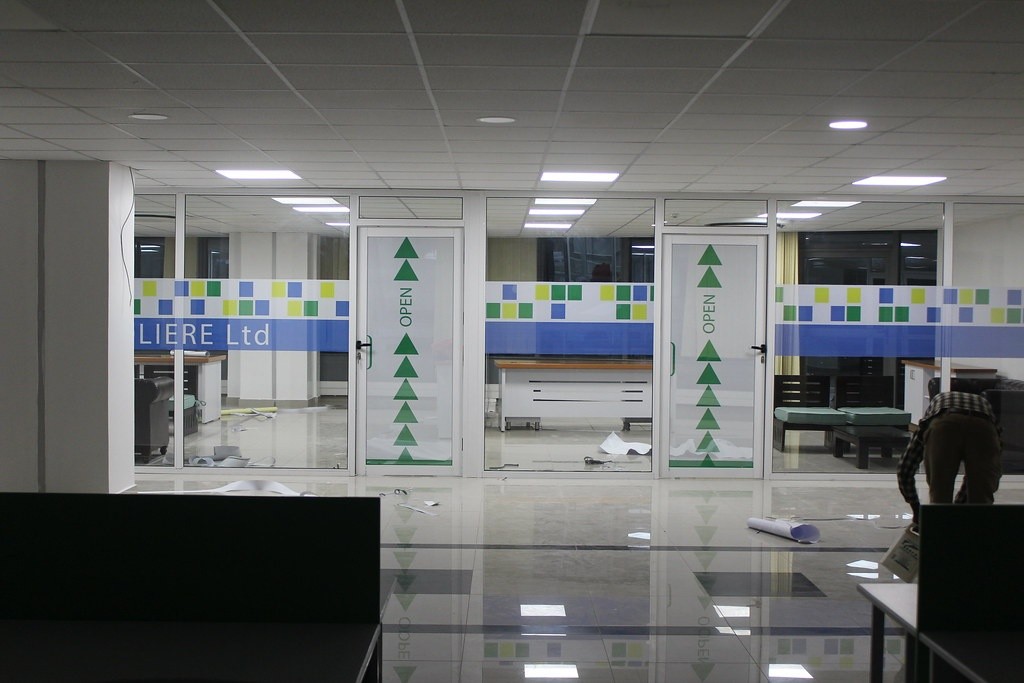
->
[493,360,653,432]
[898,359,999,430]
[135,351,227,426]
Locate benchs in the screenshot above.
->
[855,504,1024,683]
[0,490,383,683]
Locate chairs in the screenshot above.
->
[837,372,912,469]
[145,363,198,434]
[772,375,847,453]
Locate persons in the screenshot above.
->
[895,390,1006,529]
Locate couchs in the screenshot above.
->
[926,376,1024,465]
[134,377,173,466]
